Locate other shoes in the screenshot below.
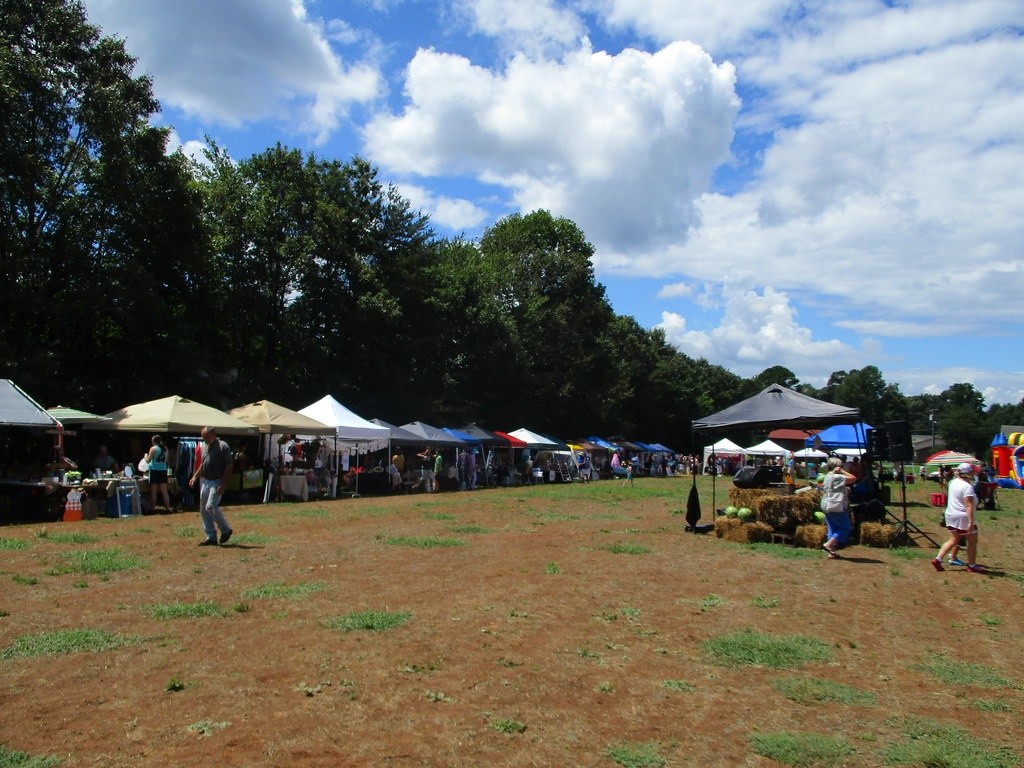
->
[219,529,233,543]
[199,538,218,546]
[431,490,439,493]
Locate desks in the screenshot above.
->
[279,476,308,503]
[1,480,96,520]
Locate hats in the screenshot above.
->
[959,463,973,473]
[578,451,584,456]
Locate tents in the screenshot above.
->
[703,438,746,477]
[564,436,675,464]
[0,378,570,497]
[744,440,788,470]
[830,448,867,463]
[792,447,829,464]
[691,383,885,536]
[804,423,873,480]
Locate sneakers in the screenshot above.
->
[967,563,985,572]
[932,558,945,572]
[949,559,965,566]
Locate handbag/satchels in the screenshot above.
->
[825,473,844,513]
[138,457,152,472]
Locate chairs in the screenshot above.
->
[864,498,885,526]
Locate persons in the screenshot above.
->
[144,435,170,514]
[92,445,119,474]
[459,450,469,473]
[931,463,996,574]
[874,461,927,484]
[188,426,233,546]
[525,456,533,476]
[820,457,856,558]
[487,451,498,488]
[705,455,863,488]
[392,446,700,493]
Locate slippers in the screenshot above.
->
[823,543,837,555]
[828,552,842,559]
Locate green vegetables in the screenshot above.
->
[65,471,81,480]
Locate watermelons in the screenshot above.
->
[725,505,753,520]
[812,511,826,524]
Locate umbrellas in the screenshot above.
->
[925,451,980,471]
